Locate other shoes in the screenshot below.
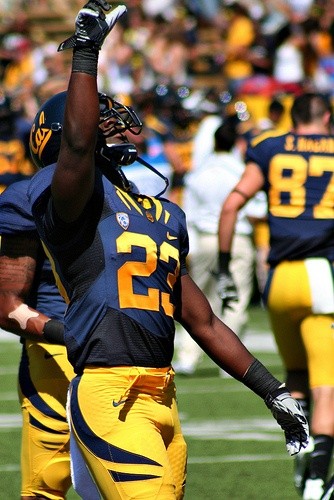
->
[173,354,198,374]
[219,367,234,379]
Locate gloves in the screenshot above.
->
[216,273,240,314]
[263,382,309,456]
[58,0,127,53]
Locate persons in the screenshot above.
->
[216,89,334,500]
[1,0,334,382]
[1,119,136,500]
[33,0,314,499]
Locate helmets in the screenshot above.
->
[29,90,143,184]
